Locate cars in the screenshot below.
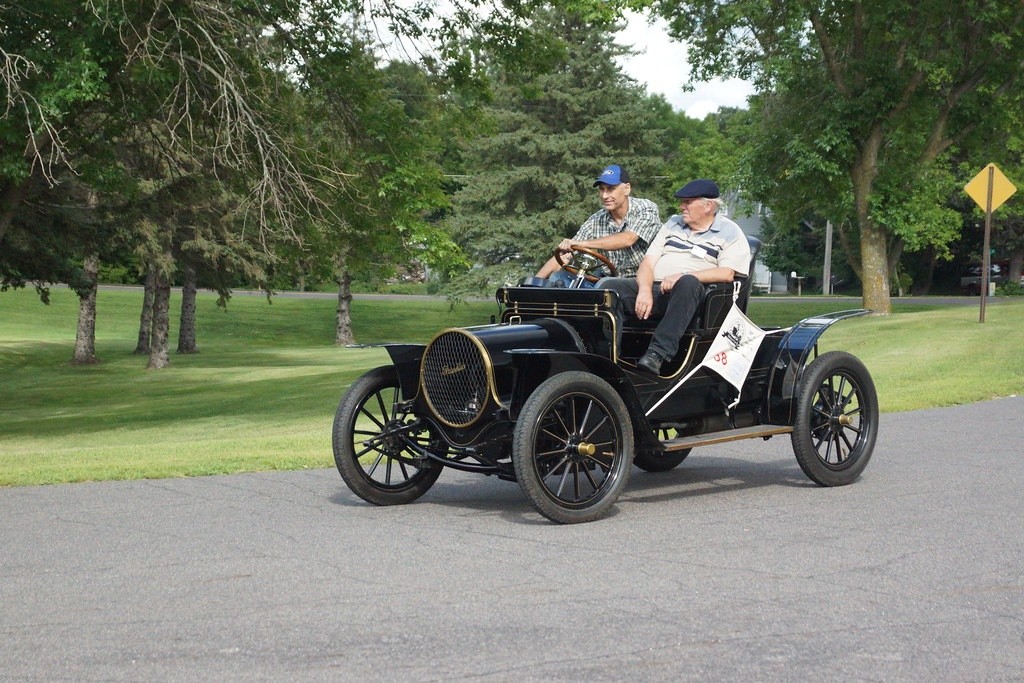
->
[960,258,1024,295]
[330,245,880,526]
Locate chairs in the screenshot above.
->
[623,234,762,335]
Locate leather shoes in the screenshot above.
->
[637,348,663,375]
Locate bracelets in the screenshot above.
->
[681,271,689,277]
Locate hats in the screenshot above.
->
[673,179,719,200]
[593,163,629,188]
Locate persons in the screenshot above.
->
[534,165,663,288]
[593,179,750,375]
